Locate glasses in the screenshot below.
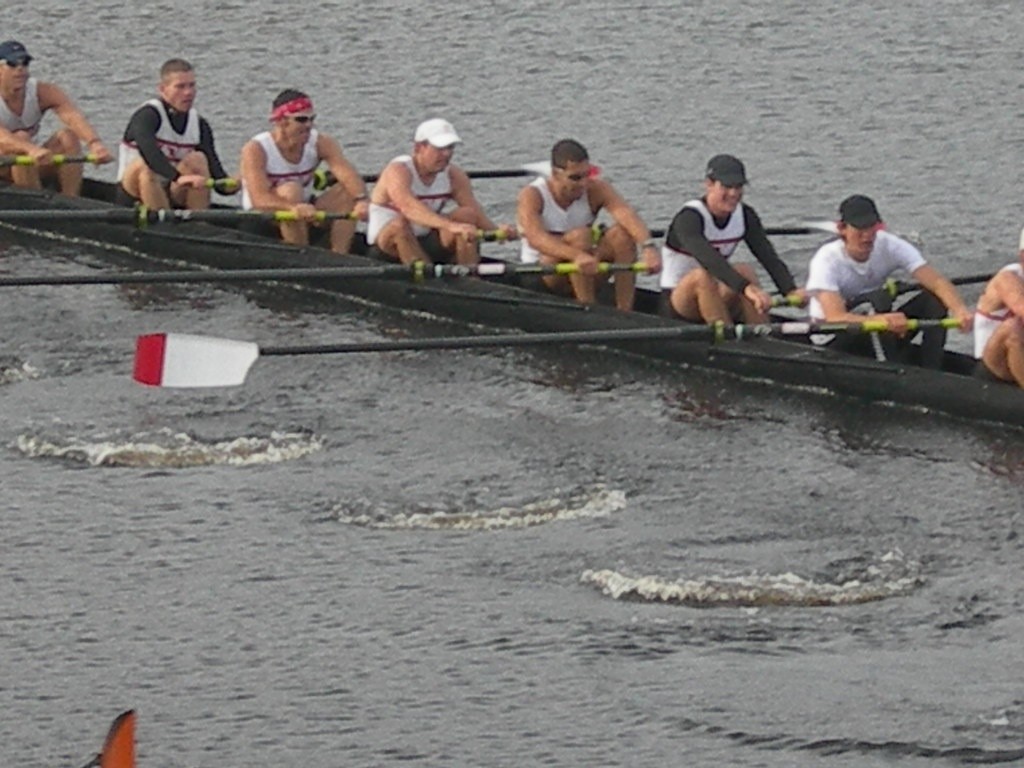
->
[285,113,318,123]
[554,167,590,181]
[0,59,30,67]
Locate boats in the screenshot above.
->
[0,164,1024,425]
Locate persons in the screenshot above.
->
[659,153,810,324]
[973,227,1024,390]
[113,58,241,210]
[805,194,974,371]
[517,138,663,312]
[363,118,520,281]
[237,89,372,255]
[0,40,115,196]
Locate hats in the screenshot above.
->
[0,41,33,60]
[706,154,748,187]
[839,195,884,229]
[414,118,464,149]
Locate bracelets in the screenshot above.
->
[353,193,369,201]
[85,136,102,148]
[640,239,657,248]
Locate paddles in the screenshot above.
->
[204,159,608,190]
[768,271,997,309]
[0,261,648,288]
[132,311,978,391]
[0,206,368,225]
[473,218,888,244]
[0,151,94,168]
